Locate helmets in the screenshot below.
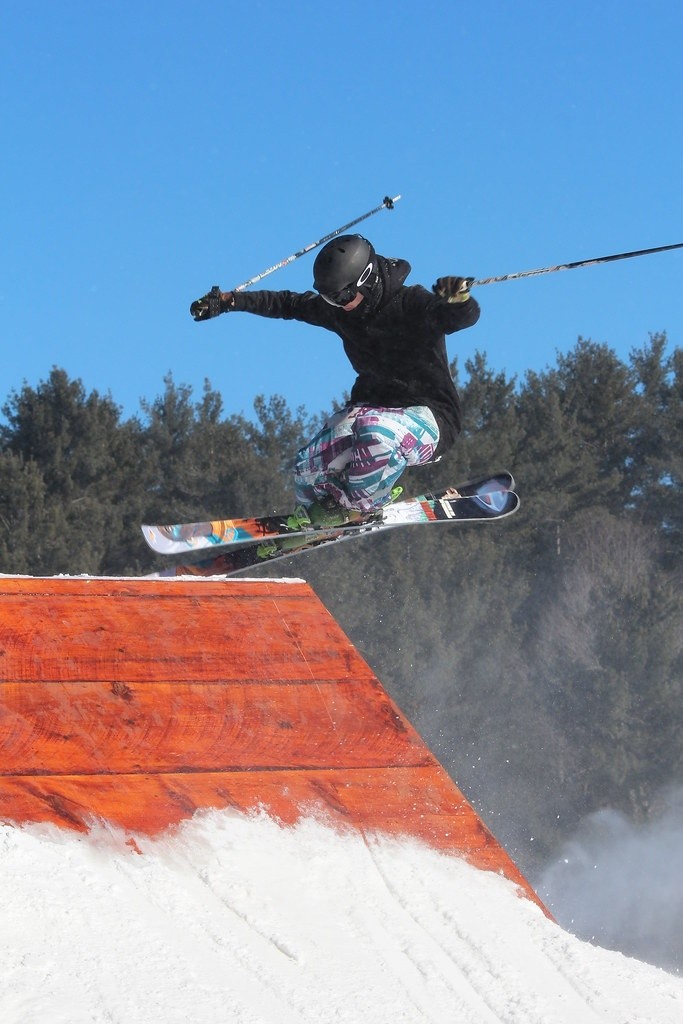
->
[313,235,370,297]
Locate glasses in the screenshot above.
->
[318,289,357,308]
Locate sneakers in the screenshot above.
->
[275,528,343,550]
[307,494,371,528]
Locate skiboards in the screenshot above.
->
[139,469,522,581]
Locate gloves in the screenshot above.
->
[190,285,235,322]
[432,276,470,304]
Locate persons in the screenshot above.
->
[189,233,482,548]
